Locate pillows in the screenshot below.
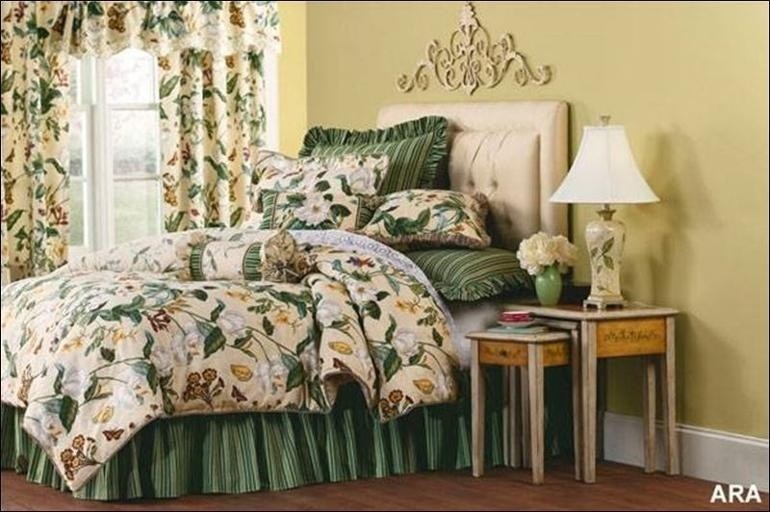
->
[174,115,529,303]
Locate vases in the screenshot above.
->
[534,263,562,306]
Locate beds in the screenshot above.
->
[1,99,570,505]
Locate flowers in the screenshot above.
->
[514,230,579,277]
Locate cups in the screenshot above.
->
[503,311,534,322]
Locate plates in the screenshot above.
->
[497,320,531,328]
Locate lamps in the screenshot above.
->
[549,115,661,309]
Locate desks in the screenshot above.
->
[504,296,680,485]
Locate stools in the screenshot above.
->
[463,329,582,486]
